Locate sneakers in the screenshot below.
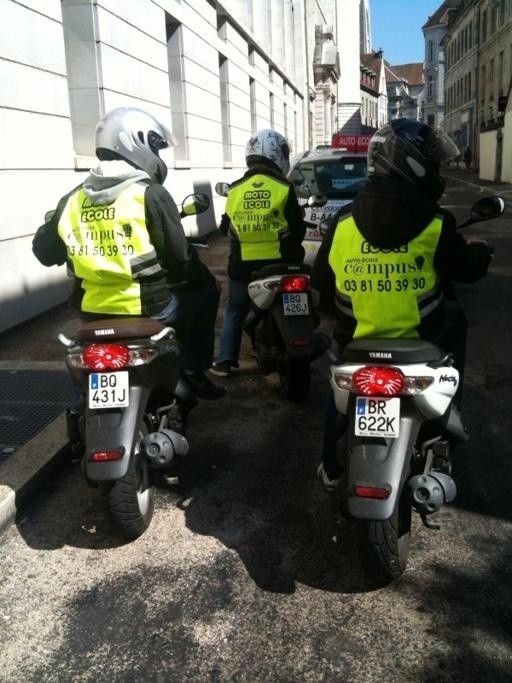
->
[66,410,83,444]
[446,408,469,446]
[317,462,340,491]
[183,373,224,399]
[209,362,232,376]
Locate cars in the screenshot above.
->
[282,133,378,270]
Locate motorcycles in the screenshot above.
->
[43,190,213,539]
[302,192,506,580]
[215,177,325,396]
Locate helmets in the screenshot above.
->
[245,129,290,178]
[95,108,169,185]
[367,117,466,201]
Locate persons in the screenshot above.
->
[463,146,472,172]
[32,106,226,459]
[209,127,304,377]
[312,118,490,488]
